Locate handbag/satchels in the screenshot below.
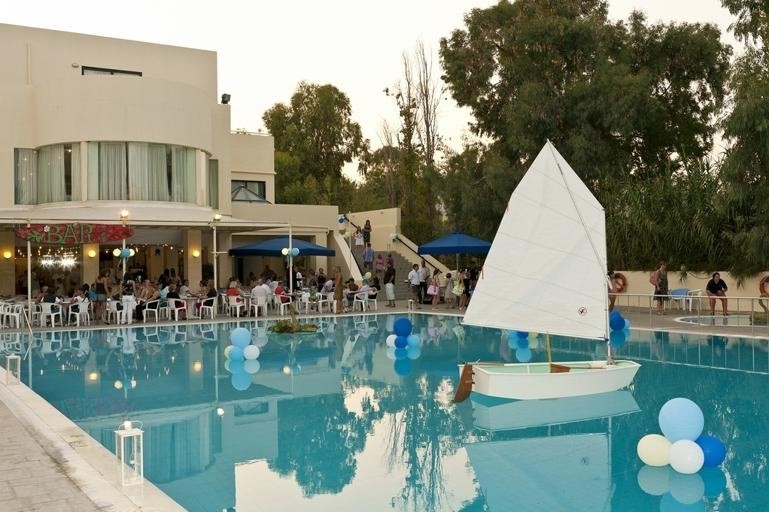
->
[426,285,440,296]
[649,269,662,287]
[451,283,465,297]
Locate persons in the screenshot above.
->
[226,265,369,317]
[705,272,729,315]
[33,265,217,324]
[355,219,396,307]
[607,270,624,312]
[652,331,669,368]
[408,259,482,311]
[652,260,669,316]
[344,316,461,376]
[706,318,728,358]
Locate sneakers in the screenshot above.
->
[384,302,468,312]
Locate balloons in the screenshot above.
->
[387,347,421,377]
[508,338,538,363]
[609,328,630,348]
[114,379,137,391]
[283,363,302,377]
[385,317,420,349]
[637,463,727,512]
[609,309,630,330]
[225,359,260,391]
[636,397,727,475]
[223,327,260,362]
[508,330,538,339]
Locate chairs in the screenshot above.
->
[664,288,701,312]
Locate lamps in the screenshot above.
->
[192,251,200,258]
[88,250,96,259]
[3,251,12,259]
[221,93,231,104]
